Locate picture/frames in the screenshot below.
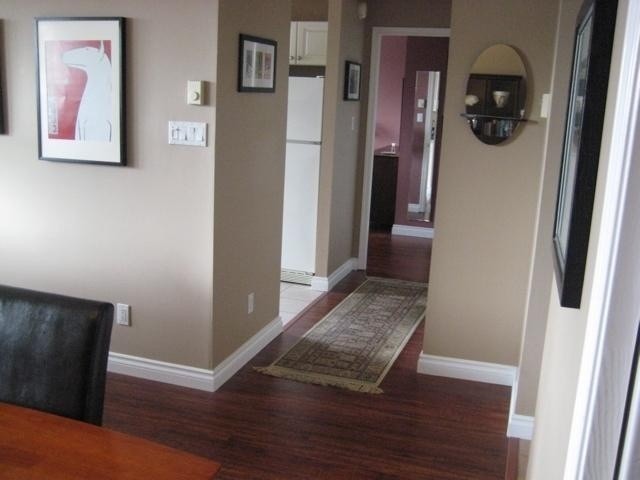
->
[237,33,277,94]
[33,15,127,168]
[548,1,620,309]
[343,57,362,102]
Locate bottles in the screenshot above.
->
[391,143,396,154]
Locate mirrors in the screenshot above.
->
[464,43,528,146]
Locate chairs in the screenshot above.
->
[0,283,115,428]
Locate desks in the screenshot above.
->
[0,403,222,480]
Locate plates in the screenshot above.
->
[382,151,395,155]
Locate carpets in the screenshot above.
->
[250,273,428,395]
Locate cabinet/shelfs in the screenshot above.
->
[289,20,328,66]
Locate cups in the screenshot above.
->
[491,91,511,108]
